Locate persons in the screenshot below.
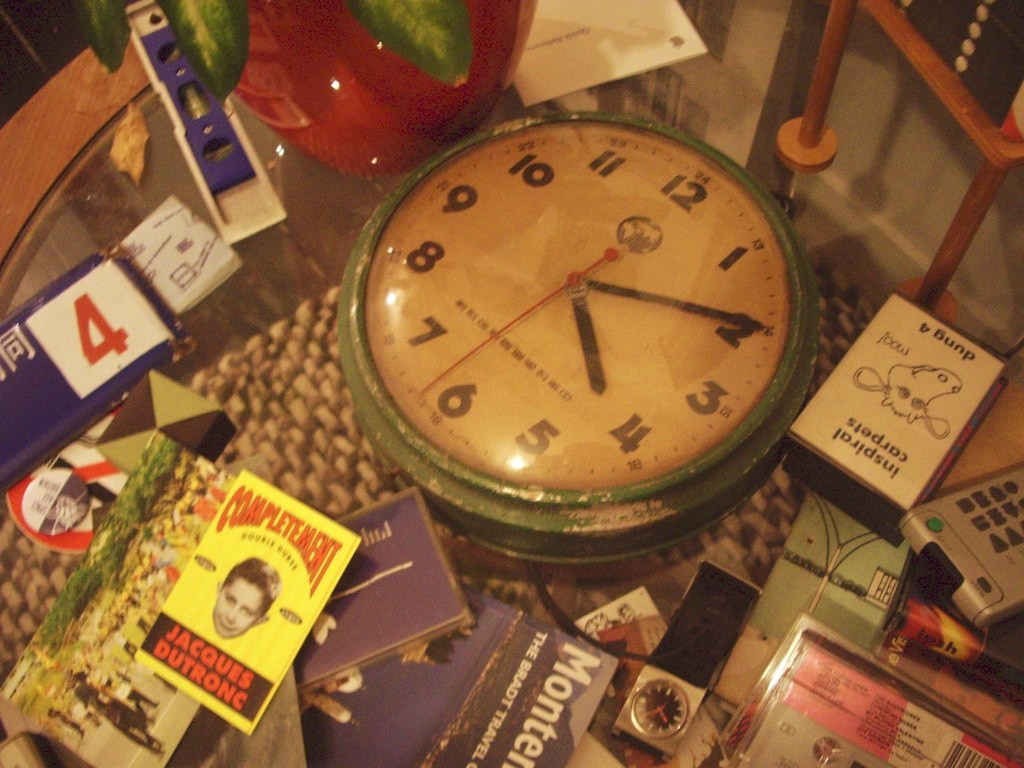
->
[65,449,234,756]
[46,707,84,737]
[213,557,280,636]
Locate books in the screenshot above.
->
[294,485,476,683]
[134,470,362,737]
[722,613,1024,767]
[296,582,619,768]
[788,292,1005,514]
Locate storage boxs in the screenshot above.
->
[785,288,1009,551]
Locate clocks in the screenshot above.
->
[334,110,822,565]
